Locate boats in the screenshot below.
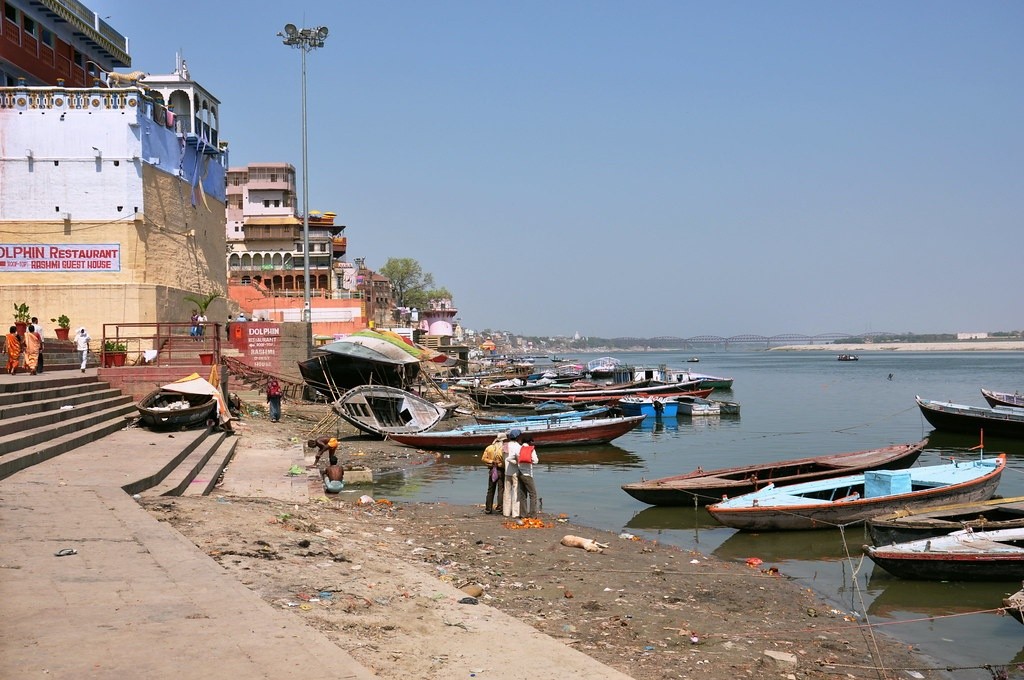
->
[703,451,1006,532]
[837,354,859,362]
[623,506,726,529]
[433,443,646,466]
[706,527,871,562]
[620,437,930,507]
[378,413,648,450]
[295,328,741,425]
[915,394,1024,435]
[867,498,1024,542]
[861,526,1024,626]
[135,371,218,434]
[331,384,447,438]
[865,577,1023,618]
[980,387,1024,411]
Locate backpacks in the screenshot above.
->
[493,443,504,467]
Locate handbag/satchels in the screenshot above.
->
[518,446,535,463]
[490,462,498,482]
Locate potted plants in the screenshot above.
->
[99,341,128,367]
[13,302,30,337]
[51,315,69,340]
[183,294,225,365]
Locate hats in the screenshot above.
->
[509,429,521,438]
[240,313,244,316]
[497,432,508,440]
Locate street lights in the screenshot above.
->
[275,23,330,322]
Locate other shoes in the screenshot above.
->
[10,372,16,375]
[81,369,85,373]
[271,419,276,423]
[29,371,37,375]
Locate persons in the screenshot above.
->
[2,317,45,375]
[306,437,338,467]
[266,377,282,423]
[322,456,344,494]
[481,429,540,518]
[189,310,265,342]
[73,328,92,373]
